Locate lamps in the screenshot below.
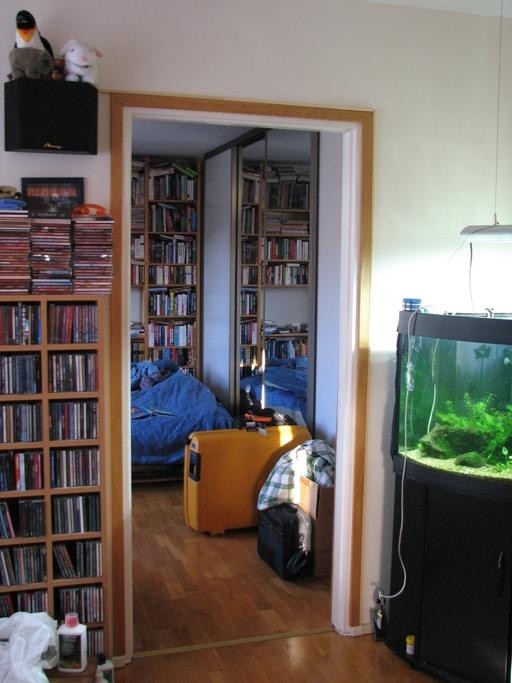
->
[459,0,511,242]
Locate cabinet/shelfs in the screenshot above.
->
[235,155,264,408]
[386,473,511,683]
[129,152,145,364]
[0,296,115,681]
[147,155,201,383]
[263,160,310,289]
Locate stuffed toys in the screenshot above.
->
[8,46,55,79]
[12,8,64,81]
[59,38,103,85]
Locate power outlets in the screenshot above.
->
[372,582,385,608]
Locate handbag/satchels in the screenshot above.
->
[257,504,315,582]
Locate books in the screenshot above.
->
[2,587,104,656]
[1,185,113,294]
[1,401,99,492]
[1,303,98,395]
[2,496,103,586]
[130,150,197,380]
[239,156,310,366]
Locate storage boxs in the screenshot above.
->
[296,474,335,581]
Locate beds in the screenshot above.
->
[127,360,231,487]
[237,356,312,431]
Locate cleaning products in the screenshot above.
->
[58,611,88,672]
[96,651,114,683]
